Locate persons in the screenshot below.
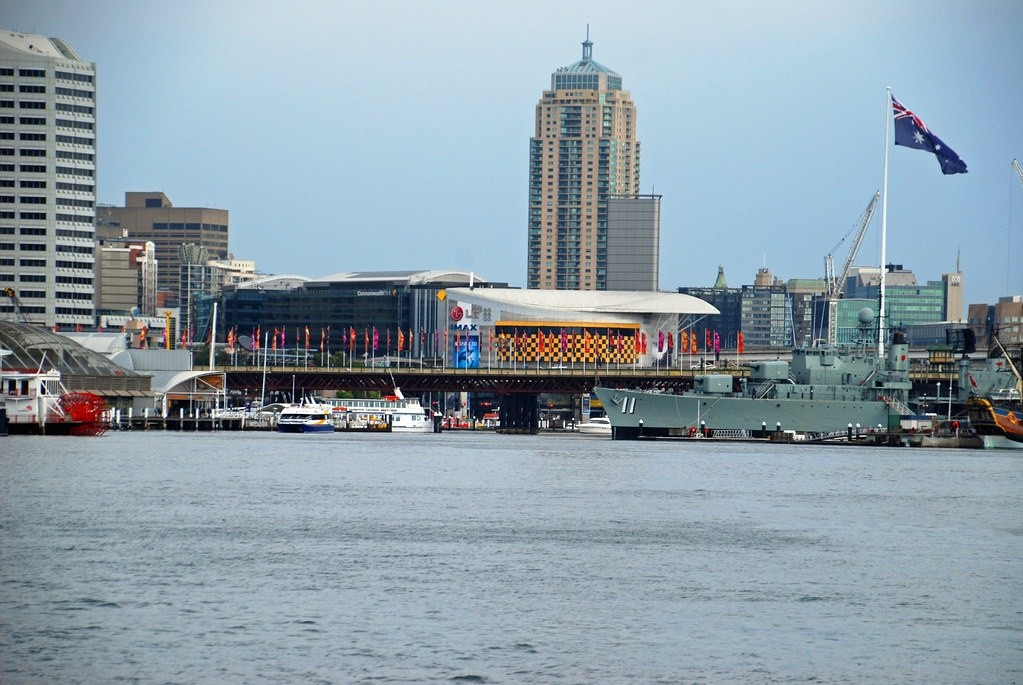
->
[139,406,211,418]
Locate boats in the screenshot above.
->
[576,417,613,434]
[566,423,578,429]
[0,369,92,436]
[965,398,1023,449]
[277,405,335,434]
[254,369,434,432]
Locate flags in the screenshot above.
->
[74,325,746,357]
[891,93,969,175]
[969,373,977,388]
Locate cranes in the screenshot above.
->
[824,189,880,349]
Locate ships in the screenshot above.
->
[592,308,923,441]
